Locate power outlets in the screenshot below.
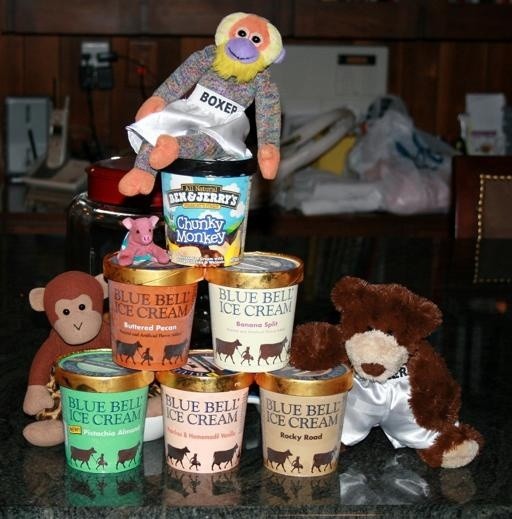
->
[77,40,113,90]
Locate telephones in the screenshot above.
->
[25,96,92,190]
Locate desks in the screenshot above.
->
[1,156,72,237]
[270,169,459,319]
[2,363,511,519]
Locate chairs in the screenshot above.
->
[421,154,512,301]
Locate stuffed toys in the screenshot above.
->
[290,279,482,469]
[118,216,169,267]
[23,270,164,445]
[121,12,287,197]
